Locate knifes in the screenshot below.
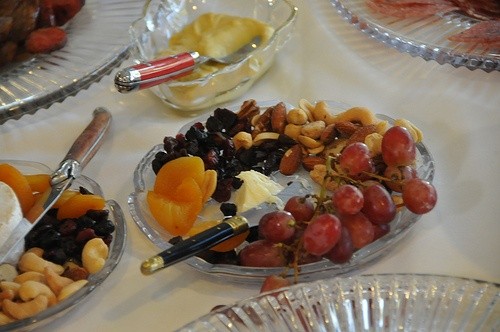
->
[138,180,306,274]
[0,106,112,262]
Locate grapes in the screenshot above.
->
[237,125,437,309]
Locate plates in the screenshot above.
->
[174,271,500,332]
[329,0,500,74]
[127,98,436,279]
[0,157,127,332]
[0,0,176,121]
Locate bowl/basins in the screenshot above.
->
[129,0,300,114]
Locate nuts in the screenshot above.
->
[0,237,111,326]
[230,99,423,176]
